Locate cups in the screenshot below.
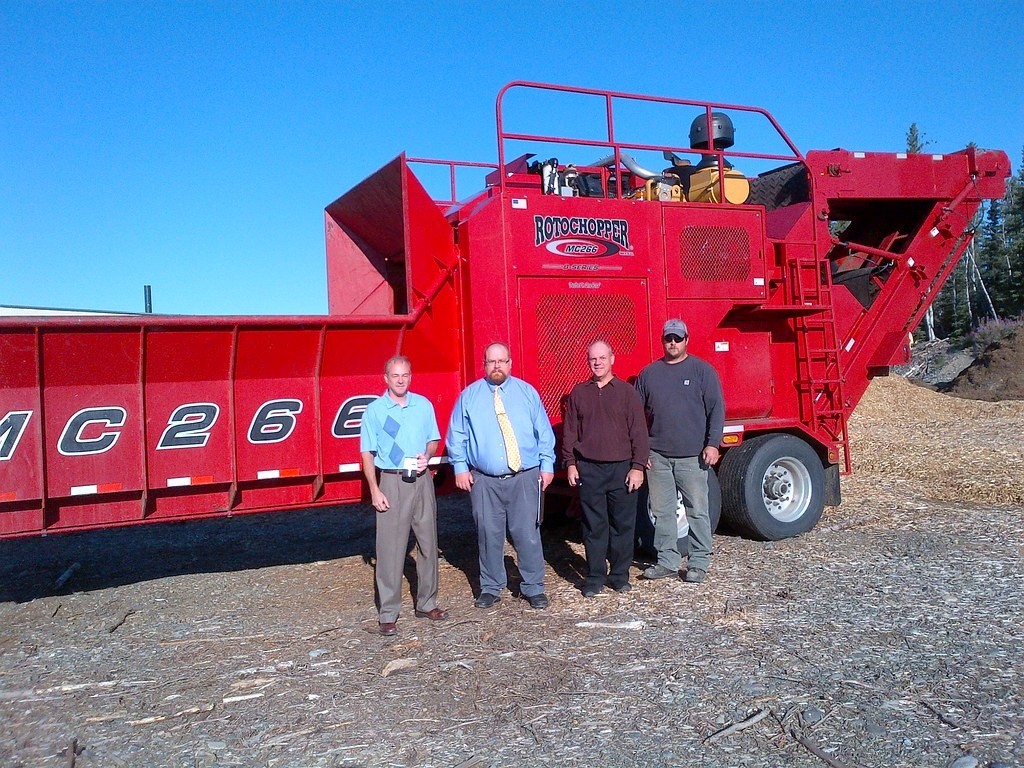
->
[402,456,420,478]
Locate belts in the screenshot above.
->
[380,469,403,475]
[472,467,539,479]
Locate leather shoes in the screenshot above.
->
[415,608,449,620]
[475,593,502,609]
[379,619,397,636]
[523,592,549,609]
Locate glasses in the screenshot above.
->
[665,336,685,343]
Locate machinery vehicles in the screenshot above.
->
[1,80,1008,541]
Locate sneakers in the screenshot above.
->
[685,567,707,582]
[581,583,604,597]
[609,581,632,592]
[642,565,678,581]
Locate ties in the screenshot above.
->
[494,389,522,472]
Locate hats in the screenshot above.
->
[663,319,688,338]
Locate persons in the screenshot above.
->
[444,340,559,610]
[562,340,650,597]
[360,356,450,636]
[633,318,726,583]
